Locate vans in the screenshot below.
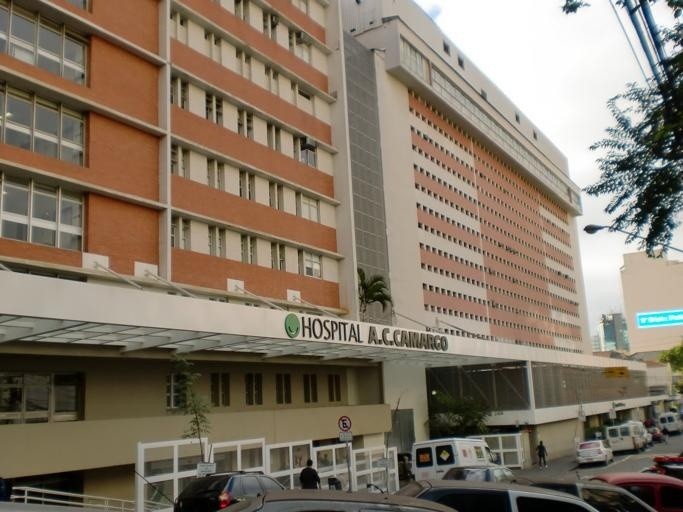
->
[606,420,652,453]
[410,437,503,480]
[658,412,683,434]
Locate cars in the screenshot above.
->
[575,440,614,466]
[647,427,666,443]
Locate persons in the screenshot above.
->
[298,459,321,491]
[652,416,660,428]
[659,425,670,446]
[398,455,414,489]
[642,417,651,429]
[534,440,548,471]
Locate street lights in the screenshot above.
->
[584,224,683,254]
